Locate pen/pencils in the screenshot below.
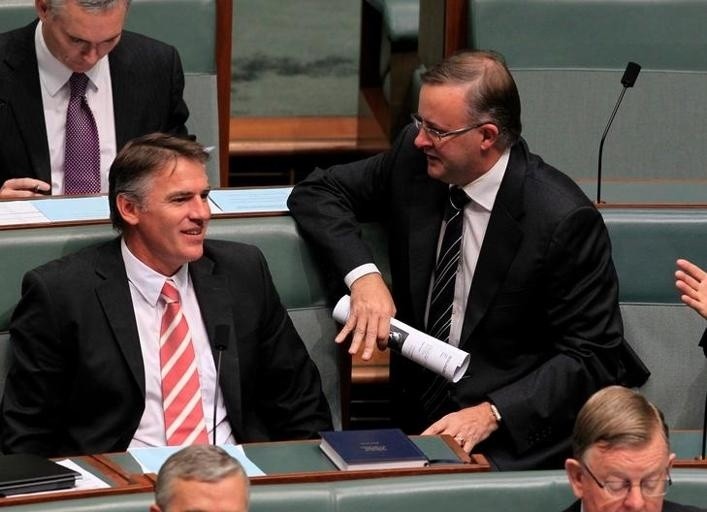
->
[34,185,39,193]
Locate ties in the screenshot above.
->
[427,186,472,344]
[160,280,209,446]
[65,72,101,195]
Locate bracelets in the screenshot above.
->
[491,405,502,421]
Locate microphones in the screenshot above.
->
[592,62,641,205]
[212,324,230,446]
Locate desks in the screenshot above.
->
[1,184,298,229]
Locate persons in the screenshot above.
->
[150,445,251,512]
[561,385,707,512]
[0,0,189,200]
[0,132,334,459]
[675,258,707,460]
[287,50,651,471]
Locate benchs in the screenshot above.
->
[2,207,707,511]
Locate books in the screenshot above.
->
[1,449,83,496]
[319,428,428,473]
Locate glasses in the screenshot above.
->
[411,113,496,142]
[575,457,672,499]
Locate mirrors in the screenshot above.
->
[217,0,462,190]
